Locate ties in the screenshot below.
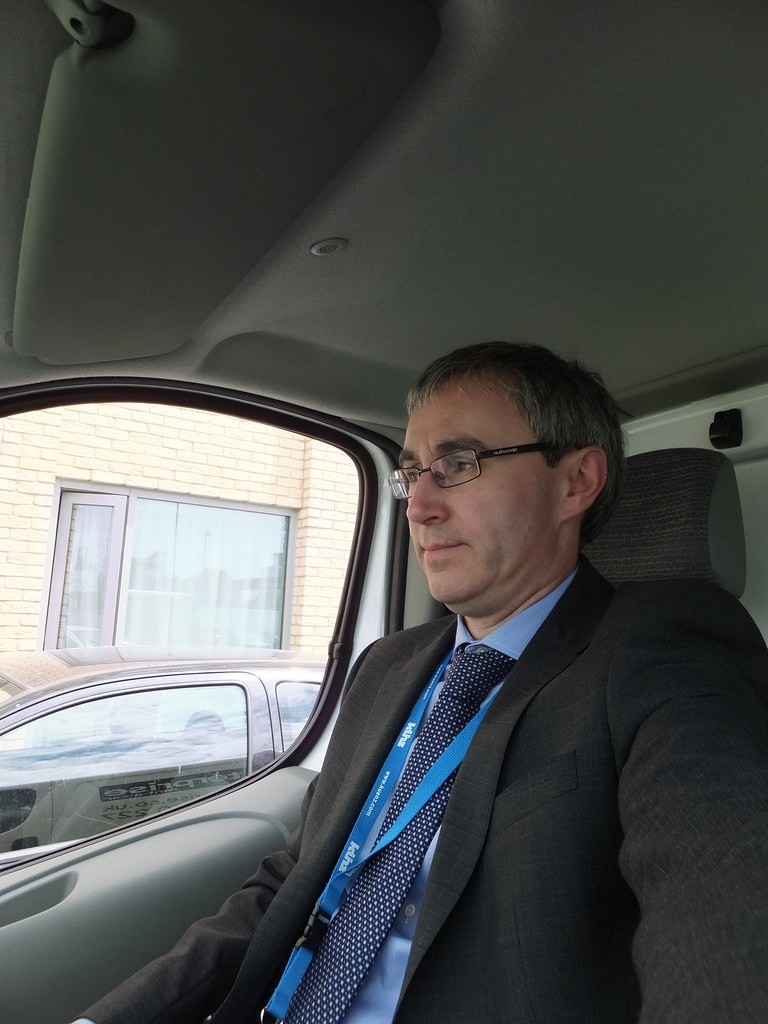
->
[279,647,516,1024]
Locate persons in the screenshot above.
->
[65,340,768,1024]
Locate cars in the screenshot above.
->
[0,643,329,874]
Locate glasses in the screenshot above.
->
[385,441,551,499]
